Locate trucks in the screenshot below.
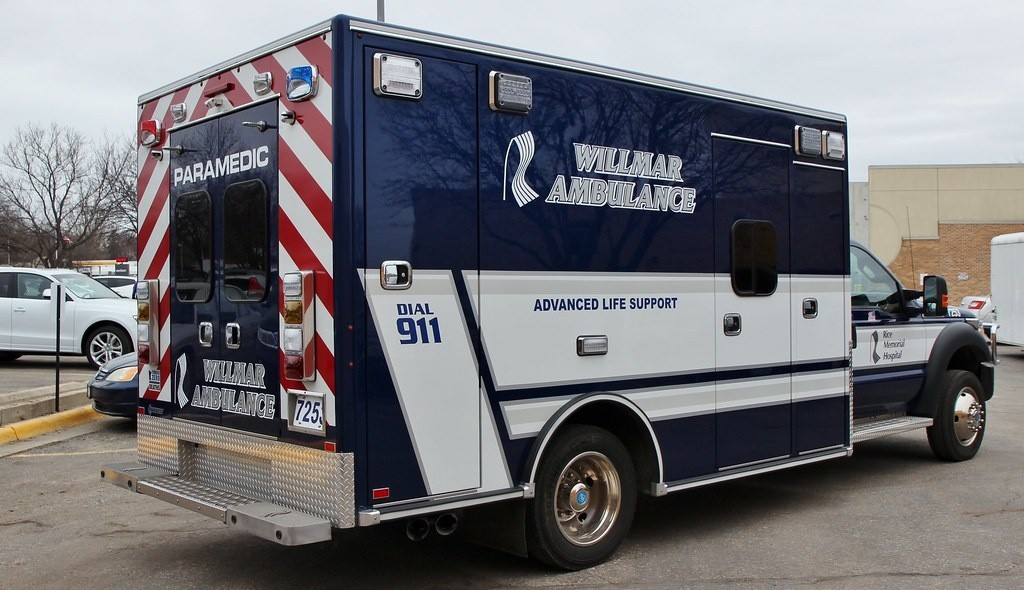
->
[100,13,1000,573]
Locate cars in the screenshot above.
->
[0,267,138,371]
[92,275,138,298]
[86,351,138,416]
[958,296,995,338]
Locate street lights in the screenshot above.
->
[7,217,32,267]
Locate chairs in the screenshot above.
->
[37,279,51,298]
[18,280,27,297]
